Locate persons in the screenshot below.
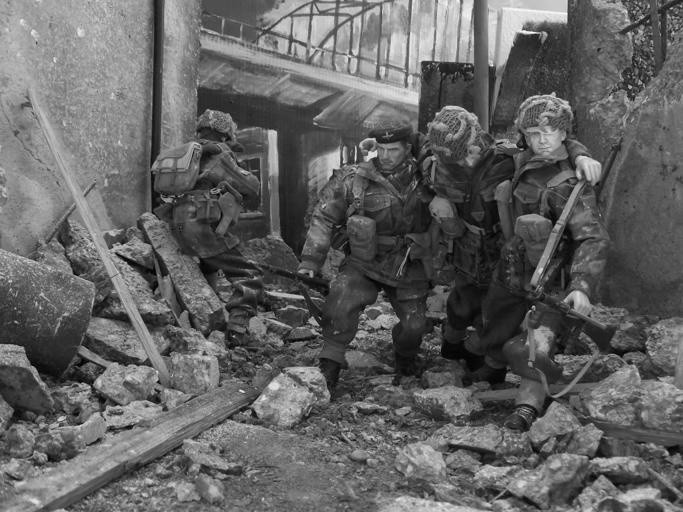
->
[294,117,430,389]
[469,91,611,432]
[357,103,603,388]
[151,107,266,352]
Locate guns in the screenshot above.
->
[526,284,617,355]
[250,259,331,296]
[542,138,623,290]
